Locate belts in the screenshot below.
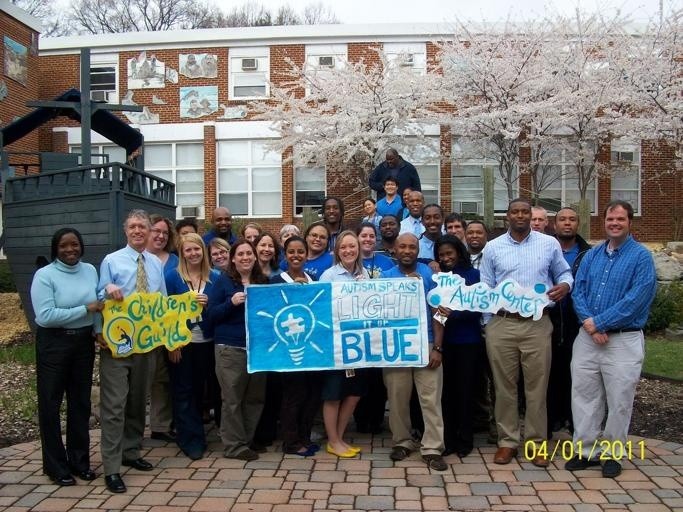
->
[62,329,87,335]
[607,328,639,333]
[496,310,547,319]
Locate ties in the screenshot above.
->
[136,254,149,294]
[414,221,420,237]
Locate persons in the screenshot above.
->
[201,54,217,77]
[368,147,422,207]
[188,100,202,117]
[199,98,212,114]
[144,176,590,472]
[29,227,108,486]
[181,55,202,76]
[96,209,168,492]
[564,200,657,477]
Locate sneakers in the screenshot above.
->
[389,432,620,478]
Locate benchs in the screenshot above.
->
[41,152,78,172]
